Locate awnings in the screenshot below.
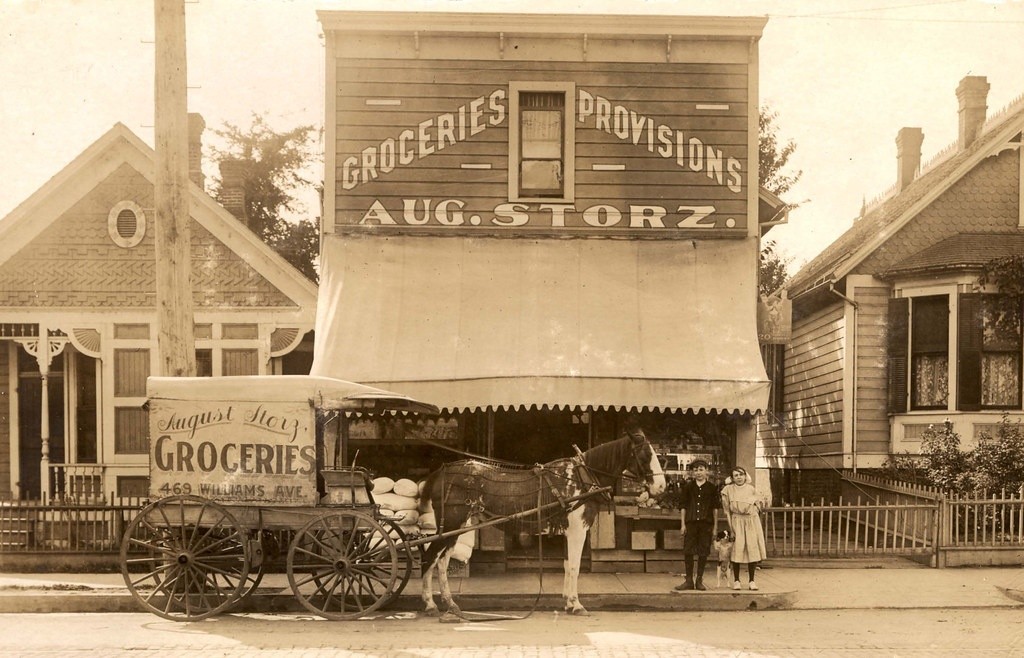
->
[308,232,774,417]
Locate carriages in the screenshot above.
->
[120,375,668,621]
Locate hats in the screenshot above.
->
[690,458,708,469]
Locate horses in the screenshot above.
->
[419,427,666,617]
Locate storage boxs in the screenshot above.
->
[664,529,685,550]
[638,507,662,516]
[615,505,639,516]
[662,508,680,515]
[631,529,659,550]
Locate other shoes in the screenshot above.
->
[749,581,758,590]
[676,577,705,591]
[733,581,741,589]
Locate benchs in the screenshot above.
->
[318,468,378,505]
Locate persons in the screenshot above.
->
[674,457,719,592]
[721,465,767,590]
